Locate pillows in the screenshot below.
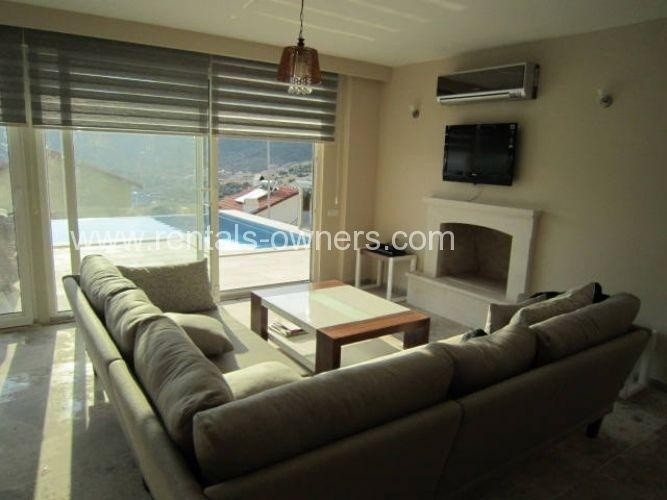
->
[118,259,221,314]
[482,280,597,337]
[222,361,305,399]
[167,309,235,357]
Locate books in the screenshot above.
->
[268,319,308,338]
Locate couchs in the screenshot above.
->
[63,256,651,500]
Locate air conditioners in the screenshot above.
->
[436,60,539,105]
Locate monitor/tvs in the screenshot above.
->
[443,123,518,186]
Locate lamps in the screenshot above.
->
[274,1,325,98]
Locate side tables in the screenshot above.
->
[354,248,418,302]
